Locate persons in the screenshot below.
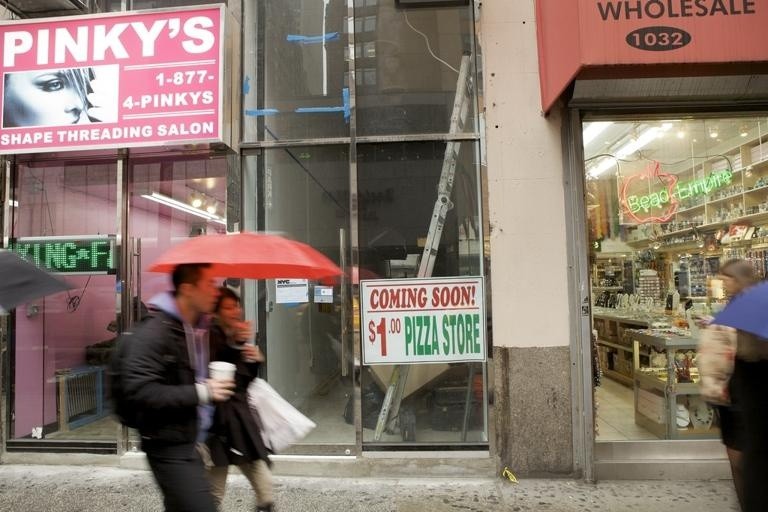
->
[119,263,237,512]
[4,68,88,127]
[708,257,758,512]
[735,330,768,512]
[205,285,277,512]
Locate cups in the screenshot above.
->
[207,362,236,402]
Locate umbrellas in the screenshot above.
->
[710,278,768,341]
[146,230,345,282]
[0,247,82,307]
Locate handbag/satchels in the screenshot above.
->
[246,377,317,455]
[696,323,739,408]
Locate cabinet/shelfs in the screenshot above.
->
[592,131,768,440]
